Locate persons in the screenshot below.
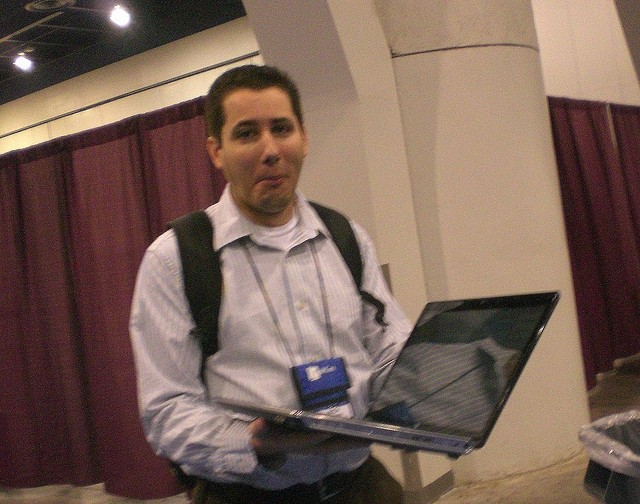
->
[129,64,428,502]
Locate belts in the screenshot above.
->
[185,475,352,504]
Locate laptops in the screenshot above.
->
[212,291,563,462]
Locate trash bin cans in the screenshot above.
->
[577,409,640,504]
